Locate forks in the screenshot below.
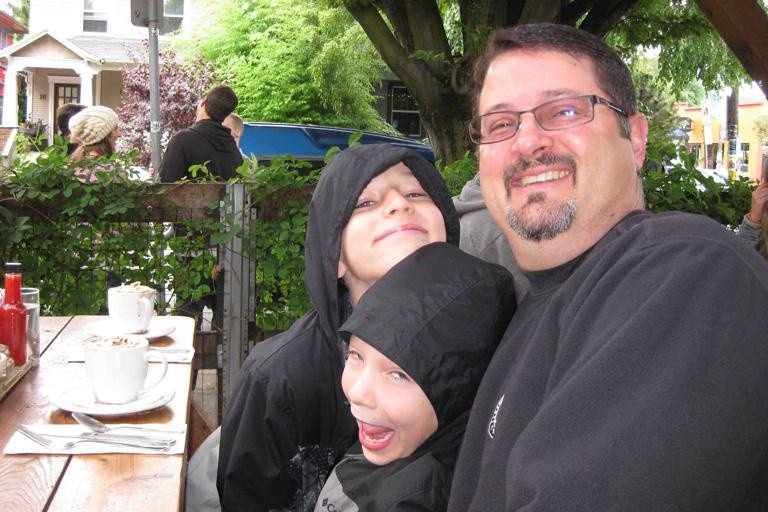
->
[15,423,169,452]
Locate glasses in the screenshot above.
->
[468,95,628,144]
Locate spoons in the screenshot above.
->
[71,412,185,434]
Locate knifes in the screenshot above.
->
[38,433,176,446]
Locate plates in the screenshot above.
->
[50,380,175,417]
[84,317,177,340]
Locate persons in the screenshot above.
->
[454,172,532,303]
[64,105,152,257]
[447,24,768,512]
[216,144,461,511]
[57,103,87,141]
[740,159,768,260]
[159,85,245,314]
[313,242,518,512]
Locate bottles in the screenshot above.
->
[0,262,27,366]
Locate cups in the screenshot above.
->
[2,287,40,367]
[84,333,168,404]
[107,284,156,334]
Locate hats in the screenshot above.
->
[68,106,119,146]
